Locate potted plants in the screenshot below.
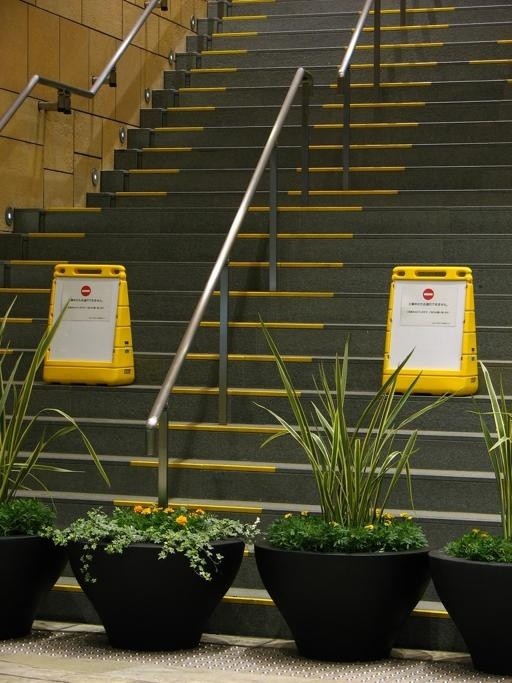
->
[0,294,109,643]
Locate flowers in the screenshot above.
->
[446,358,512,561]
[236,311,452,550]
[42,502,262,585]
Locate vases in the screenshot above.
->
[428,550,511,674]
[64,538,245,651]
[252,539,429,663]
